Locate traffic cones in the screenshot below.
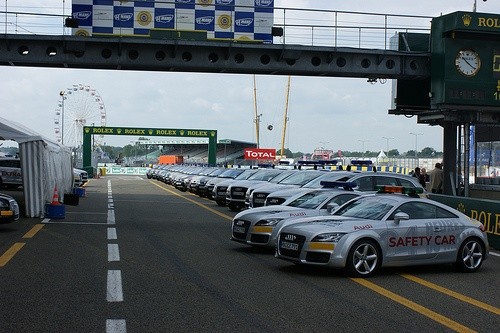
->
[51,184,59,205]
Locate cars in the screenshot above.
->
[249,160,343,211]
[226,160,290,211]
[229,181,378,255]
[146,163,229,197]
[0,157,88,191]
[212,161,273,206]
[273,185,490,278]
[264,160,428,207]
[245,160,300,209]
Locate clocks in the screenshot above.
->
[454,49,481,76]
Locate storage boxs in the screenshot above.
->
[46,187,85,219]
